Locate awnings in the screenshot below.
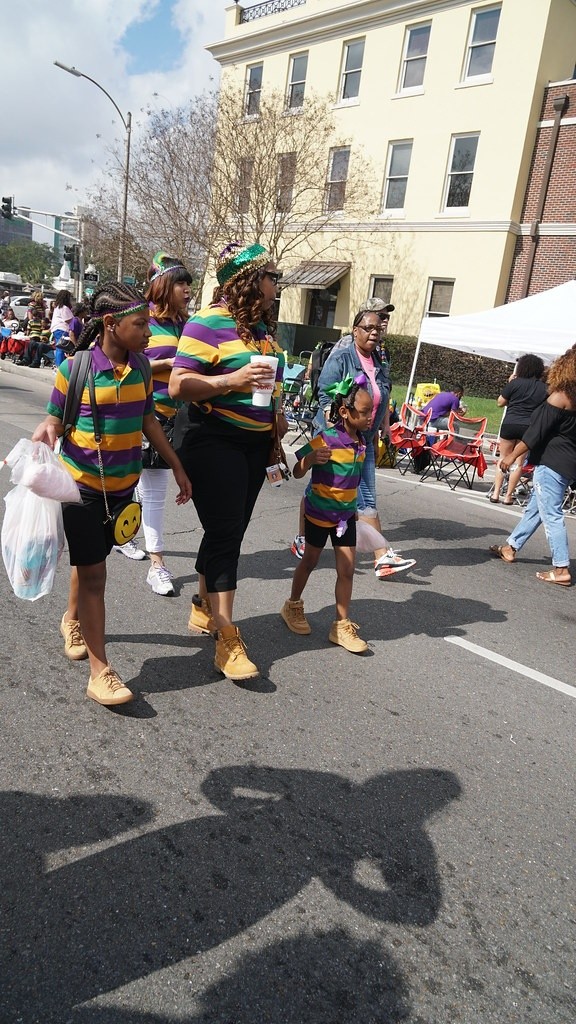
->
[277,260,350,289]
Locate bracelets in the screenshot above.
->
[304,456,311,470]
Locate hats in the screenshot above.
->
[359,298,395,312]
[216,239,272,287]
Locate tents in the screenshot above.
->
[402,279,576,456]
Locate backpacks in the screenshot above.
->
[311,343,335,401]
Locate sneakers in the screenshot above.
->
[214,625,260,680]
[188,594,218,639]
[374,548,416,577]
[87,666,133,705]
[280,599,311,634]
[145,561,175,595]
[59,611,87,660]
[112,539,146,560]
[290,534,305,559]
[329,618,368,652]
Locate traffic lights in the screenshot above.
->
[0,193,15,222]
[63,243,81,271]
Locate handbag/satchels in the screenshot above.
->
[105,499,142,546]
[1,438,83,602]
[55,336,75,352]
[141,425,174,469]
[356,519,388,554]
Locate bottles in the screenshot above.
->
[405,393,427,430]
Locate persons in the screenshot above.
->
[419,384,467,462]
[491,354,554,505]
[280,297,418,578]
[0,291,331,418]
[272,474,276,480]
[114,250,193,595]
[281,374,374,653]
[489,344,576,586]
[32,280,192,705]
[167,242,292,682]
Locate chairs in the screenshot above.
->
[281,369,321,447]
[377,402,576,514]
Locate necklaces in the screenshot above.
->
[221,297,277,358]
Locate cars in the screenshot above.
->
[0,281,59,323]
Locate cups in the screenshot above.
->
[251,355,279,407]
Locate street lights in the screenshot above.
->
[53,59,132,282]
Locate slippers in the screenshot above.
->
[486,494,498,502]
[536,570,571,586]
[489,544,512,563]
[502,501,513,505]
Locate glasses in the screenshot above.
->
[357,325,384,333]
[377,313,390,321]
